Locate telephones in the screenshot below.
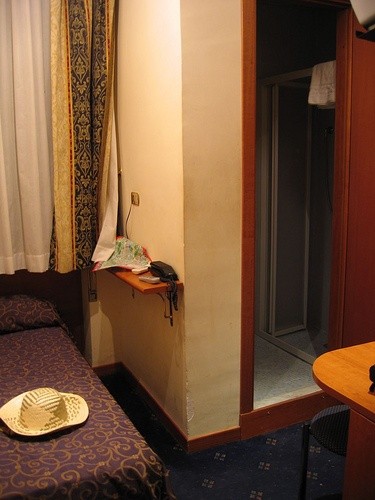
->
[150,261,178,282]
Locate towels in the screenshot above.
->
[308,60,336,105]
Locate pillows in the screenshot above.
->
[0,293,72,336]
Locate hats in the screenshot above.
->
[0,385,89,438]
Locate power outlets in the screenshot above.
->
[130,192,139,206]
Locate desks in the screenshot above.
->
[313,341,375,500]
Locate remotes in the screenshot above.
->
[139,276,160,283]
[132,267,148,275]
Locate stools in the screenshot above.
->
[298,404,351,500]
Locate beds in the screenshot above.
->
[0,266,177,500]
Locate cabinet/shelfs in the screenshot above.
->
[106,266,183,294]
